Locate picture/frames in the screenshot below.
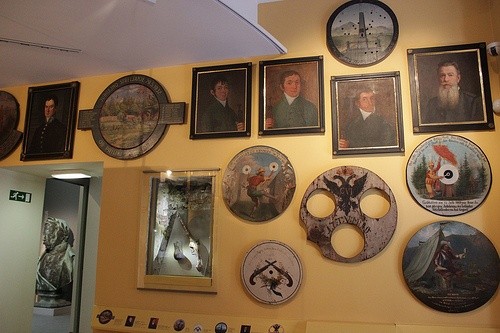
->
[136,168,223,293]
[240,239,302,306]
[257,54,327,136]
[19,79,82,163]
[405,133,495,216]
[324,1,400,68]
[330,71,405,157]
[220,145,298,223]
[189,61,254,141]
[405,41,491,134]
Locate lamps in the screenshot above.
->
[49,169,94,179]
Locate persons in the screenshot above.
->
[339,88,397,148]
[265,71,318,129]
[29,96,64,153]
[36,216,75,306]
[424,61,486,123]
[433,240,466,295]
[197,77,245,133]
[425,157,445,198]
[247,167,282,218]
